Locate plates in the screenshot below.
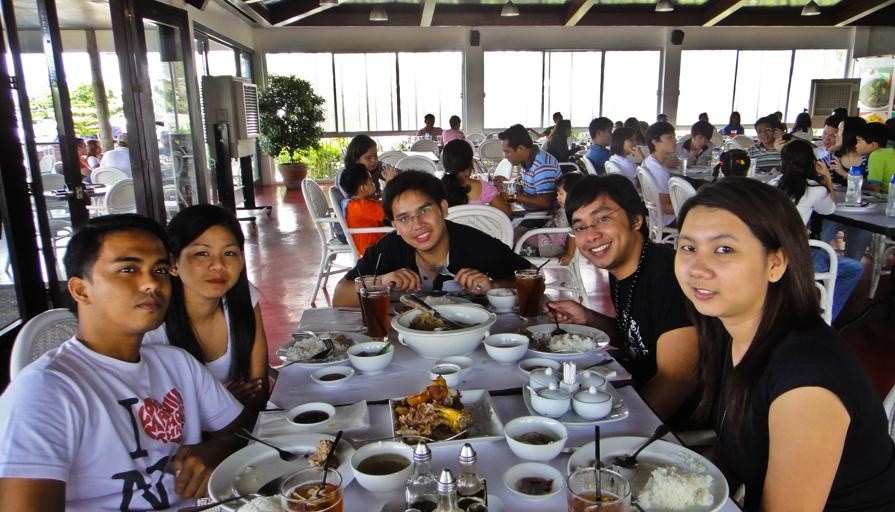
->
[208,332,373,512]
[522,370,630,425]
[519,323,611,374]
[503,436,729,511]
[389,389,507,447]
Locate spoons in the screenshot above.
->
[612,424,672,468]
[546,301,568,336]
[177,473,288,511]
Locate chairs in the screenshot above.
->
[1,132,895,383]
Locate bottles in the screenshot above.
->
[710,147,722,177]
[845,165,863,204]
[406,436,487,512]
[885,174,895,217]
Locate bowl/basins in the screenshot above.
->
[349,441,414,494]
[348,341,394,375]
[430,333,530,387]
[504,367,612,461]
[487,287,518,313]
[390,305,497,361]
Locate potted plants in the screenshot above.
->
[257,73,328,191]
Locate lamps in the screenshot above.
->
[501,1,520,17]
[655,2,675,12]
[367,7,390,20]
[801,0,822,17]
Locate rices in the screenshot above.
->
[287,337,324,359]
[424,295,454,306]
[549,333,596,351]
[637,458,713,510]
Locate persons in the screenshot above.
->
[74,128,171,185]
[544,109,895,512]
[139,204,270,422]
[333,112,577,307]
[0,214,249,511]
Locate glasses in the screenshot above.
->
[566,206,621,238]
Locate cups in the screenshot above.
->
[730,129,738,138]
[515,268,544,318]
[750,158,756,175]
[675,155,688,173]
[354,276,392,340]
[503,181,516,193]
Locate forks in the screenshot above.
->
[429,264,484,290]
